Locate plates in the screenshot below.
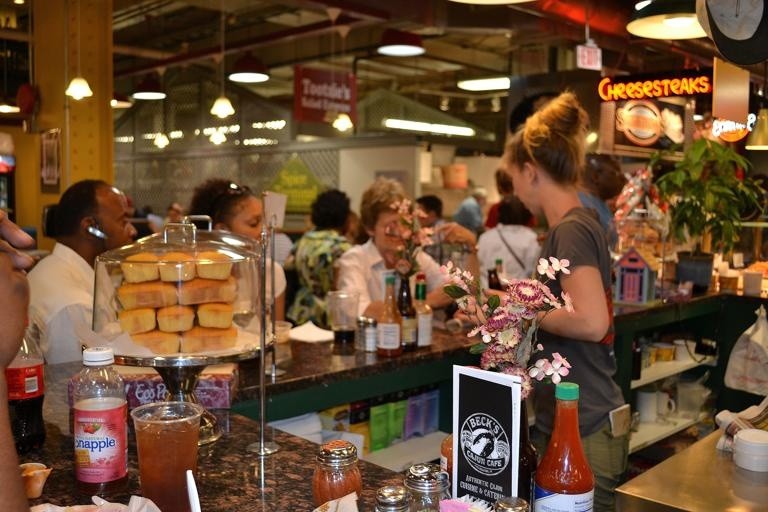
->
[732,428,768,473]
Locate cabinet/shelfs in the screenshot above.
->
[627,339,719,454]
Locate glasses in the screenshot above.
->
[224,183,251,195]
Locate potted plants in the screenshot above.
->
[644,132,768,296]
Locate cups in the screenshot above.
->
[128,401,205,511]
[721,271,763,298]
[325,290,360,346]
[641,339,697,366]
[273,320,293,343]
[637,382,705,429]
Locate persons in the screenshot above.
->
[572,151,629,256]
[160,202,186,242]
[287,168,547,326]
[456,92,630,511]
[124,198,157,247]
[184,176,289,334]
[7,178,140,364]
[140,206,166,234]
[1,210,38,512]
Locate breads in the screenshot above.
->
[194,252,233,280]
[118,309,157,336]
[180,325,240,353]
[121,252,159,284]
[178,276,239,307]
[196,304,235,328]
[157,305,195,332]
[142,330,180,353]
[158,252,195,282]
[116,282,178,310]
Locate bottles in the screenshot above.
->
[311,439,453,512]
[354,315,378,352]
[4,315,51,453]
[531,383,596,512]
[67,344,133,499]
[375,272,436,356]
[483,257,504,293]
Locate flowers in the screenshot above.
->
[439,255,576,402]
[384,198,435,279]
